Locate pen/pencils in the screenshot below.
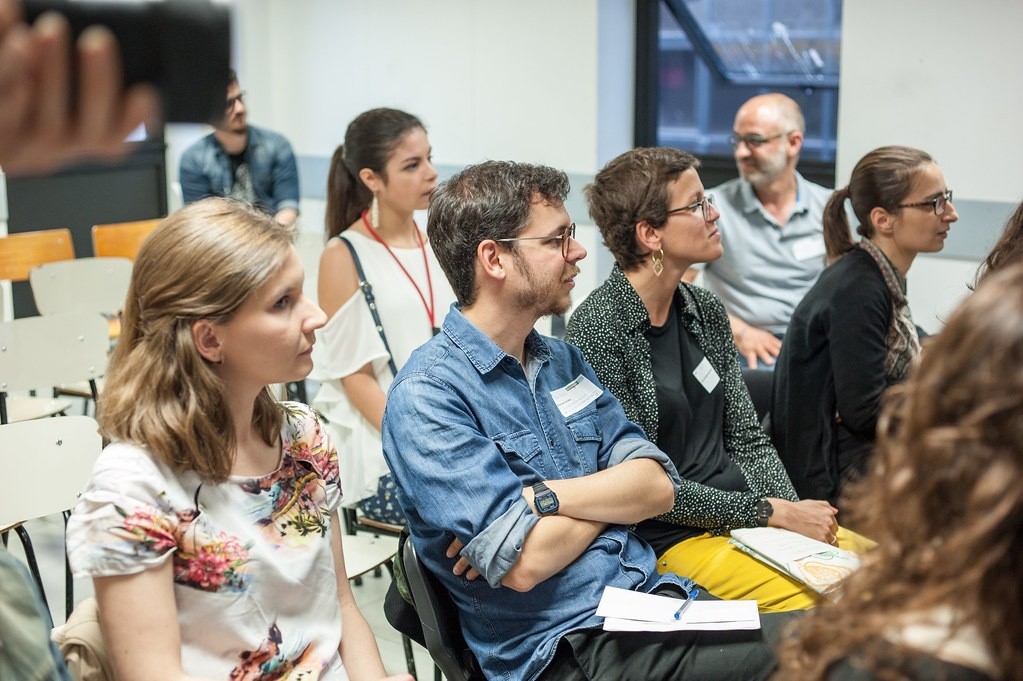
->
[674,589,700,620]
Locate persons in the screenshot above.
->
[313,106,463,537]
[0,0,159,174]
[64,194,410,680]
[382,91,1023,680]
[179,66,300,229]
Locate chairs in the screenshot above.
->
[0,218,488,681]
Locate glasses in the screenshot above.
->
[888,190,953,216]
[727,130,794,151]
[495,223,577,259]
[633,194,717,232]
[226,92,245,114]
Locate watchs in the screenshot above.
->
[530,476,561,517]
[755,497,773,528]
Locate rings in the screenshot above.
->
[831,534,837,544]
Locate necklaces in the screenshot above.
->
[358,204,434,329]
